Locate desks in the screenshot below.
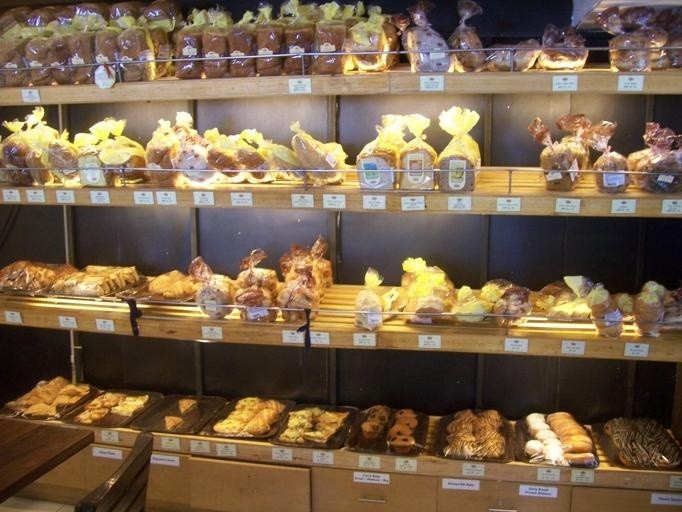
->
[0,414,94,510]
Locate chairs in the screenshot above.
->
[77,429,154,511]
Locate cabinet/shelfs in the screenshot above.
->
[0,35,681,510]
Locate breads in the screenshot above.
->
[0,0,682,471]
